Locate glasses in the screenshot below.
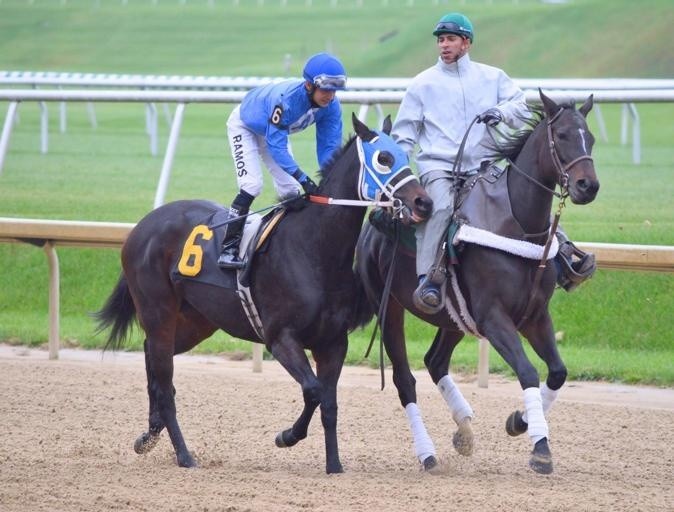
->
[436,21,471,33]
[313,73,347,89]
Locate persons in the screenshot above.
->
[215,52,348,267]
[390,12,597,308]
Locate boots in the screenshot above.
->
[215,204,249,268]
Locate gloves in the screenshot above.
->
[292,168,320,196]
[475,108,502,128]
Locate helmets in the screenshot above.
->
[433,12,474,44]
[302,52,348,91]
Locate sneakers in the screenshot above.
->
[418,277,441,306]
[556,253,596,293]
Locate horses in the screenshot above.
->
[92,111,435,476]
[352,88,601,476]
